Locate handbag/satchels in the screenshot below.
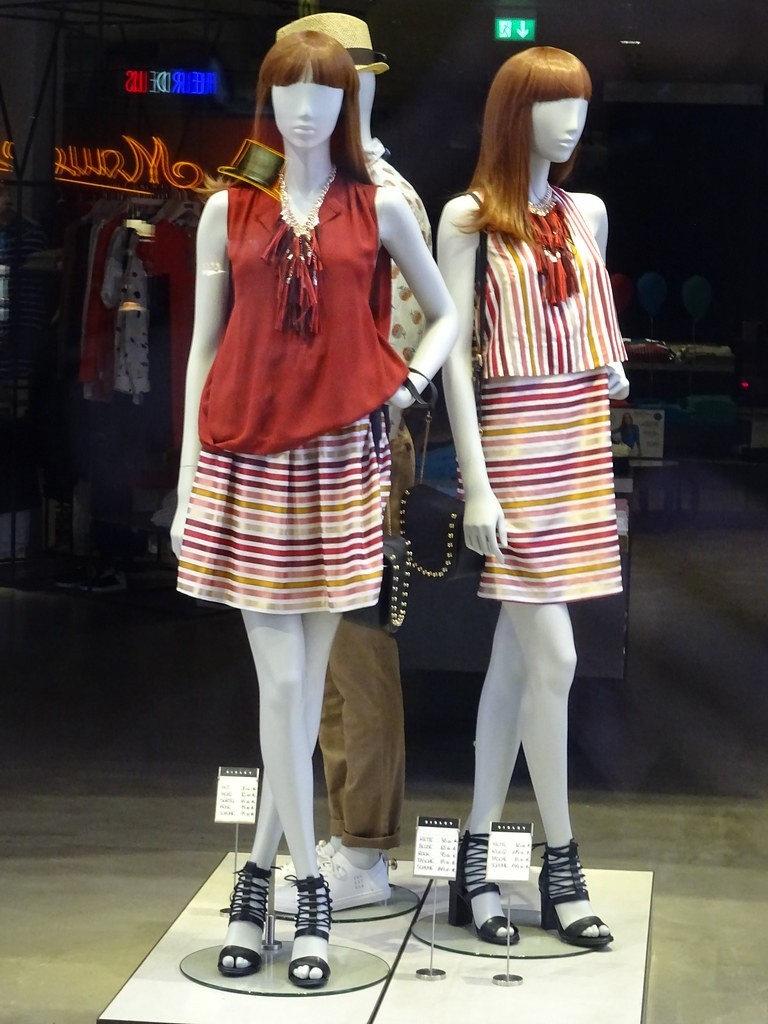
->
[343,534,412,635]
[397,483,464,580]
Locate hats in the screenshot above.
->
[276,13,388,76]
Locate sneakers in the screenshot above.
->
[265,839,391,913]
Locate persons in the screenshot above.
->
[611,412,642,458]
[166,12,461,993]
[435,42,631,949]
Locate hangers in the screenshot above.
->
[0,184,38,226]
[80,180,202,233]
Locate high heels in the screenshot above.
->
[217,862,272,977]
[531,838,613,946]
[448,829,520,946]
[285,873,333,987]
